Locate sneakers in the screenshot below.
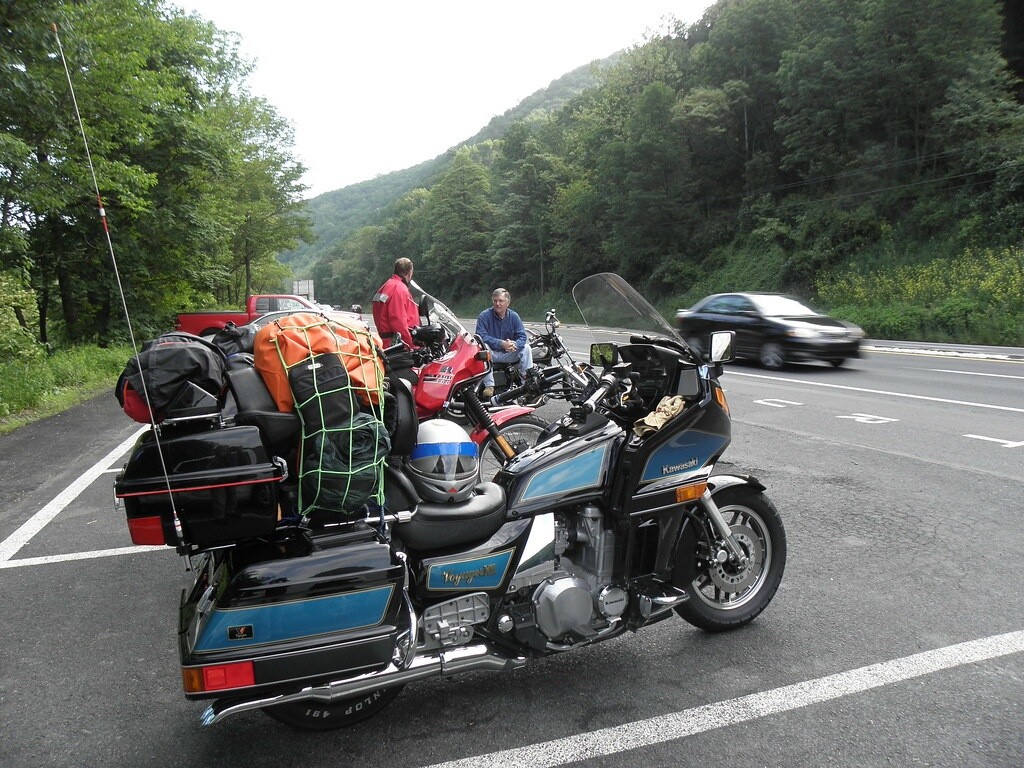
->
[483,387,494,397]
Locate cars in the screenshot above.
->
[671,291,866,372]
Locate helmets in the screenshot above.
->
[402,418,480,504]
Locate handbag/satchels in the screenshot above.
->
[289,353,360,435]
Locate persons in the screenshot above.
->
[474,288,533,397]
[371,256,423,350]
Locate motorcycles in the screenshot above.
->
[48,20,787,724]
